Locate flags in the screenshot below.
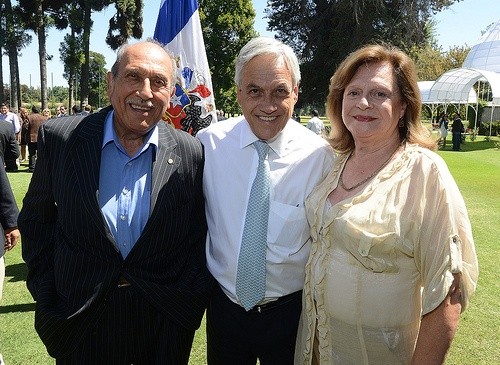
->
[153,0,218,138]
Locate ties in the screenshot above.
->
[235,141,271,312]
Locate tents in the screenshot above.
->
[417,67,500,143]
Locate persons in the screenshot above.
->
[216,109,226,121]
[0,102,92,173]
[194,36,338,365]
[18,37,208,365]
[292,108,325,135]
[294,39,479,365]
[437,113,464,151]
[0,156,20,300]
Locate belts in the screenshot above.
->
[213,286,303,312]
[118,277,131,288]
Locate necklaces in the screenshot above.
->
[339,142,401,191]
